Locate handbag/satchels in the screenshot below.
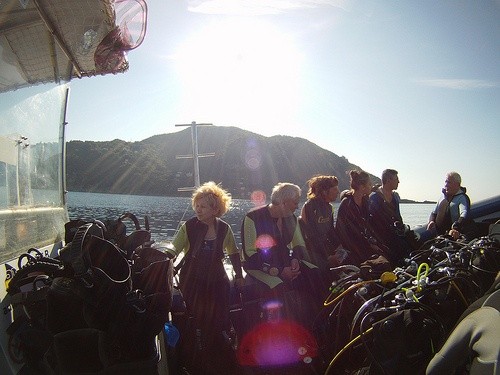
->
[372,307,429,375]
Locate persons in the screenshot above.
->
[242,183,319,330]
[426,273,500,375]
[302,169,417,273]
[164,181,245,362]
[428,171,471,239]
